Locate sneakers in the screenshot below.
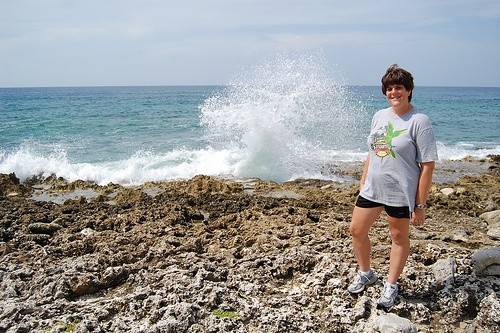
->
[348,268,378,293]
[379,283,399,307]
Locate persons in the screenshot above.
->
[345,63,438,308]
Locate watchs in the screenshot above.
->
[414,203,427,210]
